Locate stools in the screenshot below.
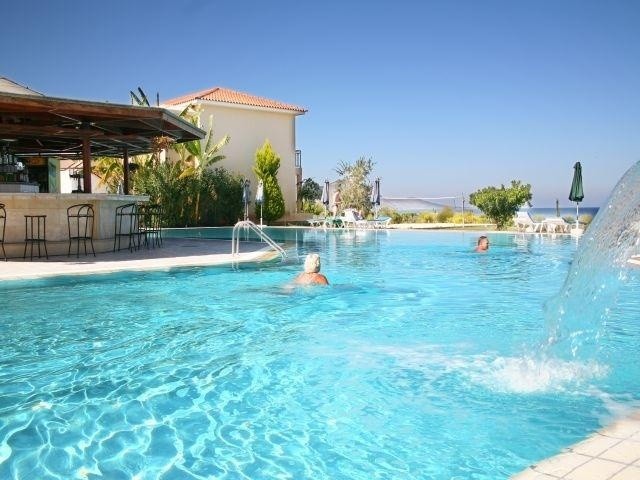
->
[23,215,48,262]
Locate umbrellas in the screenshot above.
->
[370,177,381,225]
[255,179,265,225]
[568,161,585,228]
[321,177,330,225]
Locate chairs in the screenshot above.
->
[113,203,164,253]
[0,203,7,262]
[66,203,97,259]
[514,211,570,234]
[306,215,392,229]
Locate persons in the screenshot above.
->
[293,253,329,289]
[346,204,365,219]
[331,189,344,228]
[474,235,489,254]
